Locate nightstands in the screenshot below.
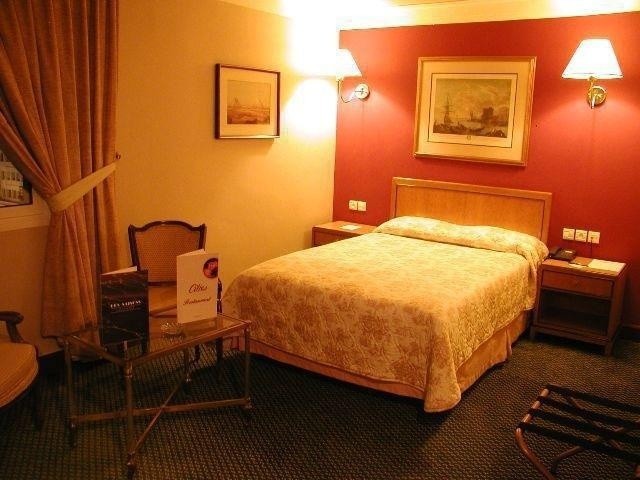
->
[311,221,377,246]
[531,255,630,355]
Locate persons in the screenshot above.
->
[205,260,218,277]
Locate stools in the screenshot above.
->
[514,381,637,480]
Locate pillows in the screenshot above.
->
[373,214,544,260]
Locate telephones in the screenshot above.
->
[548,246,577,260]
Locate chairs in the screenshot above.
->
[128,219,207,374]
[0,311,43,433]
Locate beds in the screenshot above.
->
[220,176,553,413]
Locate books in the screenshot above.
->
[176,248,220,324]
[587,259,627,276]
[100,266,150,346]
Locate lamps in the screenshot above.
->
[560,38,623,110]
[319,49,371,103]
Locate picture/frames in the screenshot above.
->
[411,54,538,167]
[215,62,281,140]
